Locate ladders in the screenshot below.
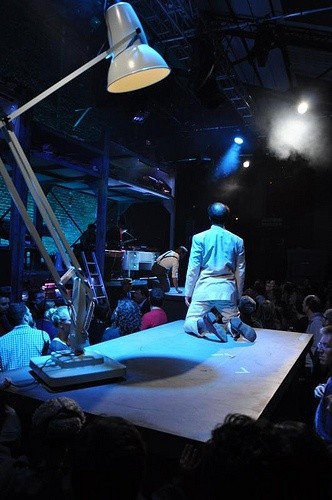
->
[82,251,112,318]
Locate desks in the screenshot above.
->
[105,249,157,278]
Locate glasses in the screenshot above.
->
[317,342,332,349]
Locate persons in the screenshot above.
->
[184,202,257,342]
[0,396,332,500]
[239,278,332,449]
[80,224,96,272]
[107,221,125,250]
[0,275,168,372]
[151,246,188,293]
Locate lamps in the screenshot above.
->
[0,2,171,389]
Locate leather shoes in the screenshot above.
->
[203,312,227,342]
[230,318,257,342]
